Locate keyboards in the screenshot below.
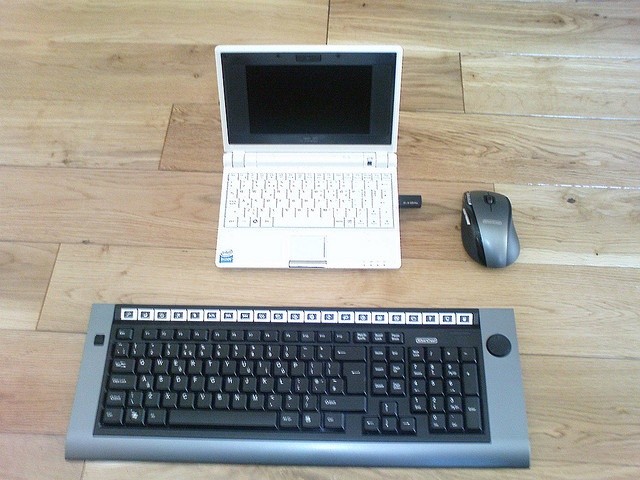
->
[64,302,530,469]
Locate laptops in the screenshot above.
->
[214,44,403,270]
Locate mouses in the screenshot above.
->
[461,190,521,268]
